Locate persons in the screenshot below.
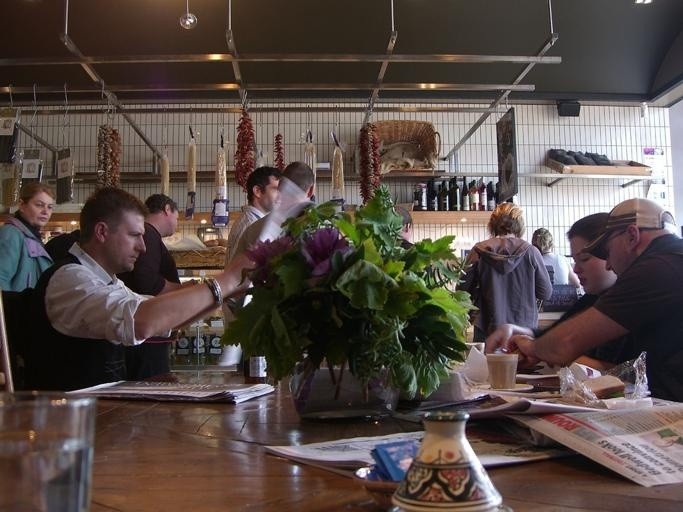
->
[227,162,315,309]
[29,186,259,392]
[216,166,283,367]
[481,213,646,380]
[531,226,585,299]
[117,193,203,378]
[455,202,553,348]
[500,195,682,402]
[0,180,56,291]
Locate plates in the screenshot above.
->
[476,383,534,393]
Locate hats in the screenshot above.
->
[585,198,676,259]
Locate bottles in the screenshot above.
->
[244,343,267,384]
[411,176,498,211]
[390,409,503,509]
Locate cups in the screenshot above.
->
[0,392,98,512]
[351,464,399,509]
[485,353,519,389]
[464,342,484,366]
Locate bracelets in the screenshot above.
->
[204,277,224,305]
